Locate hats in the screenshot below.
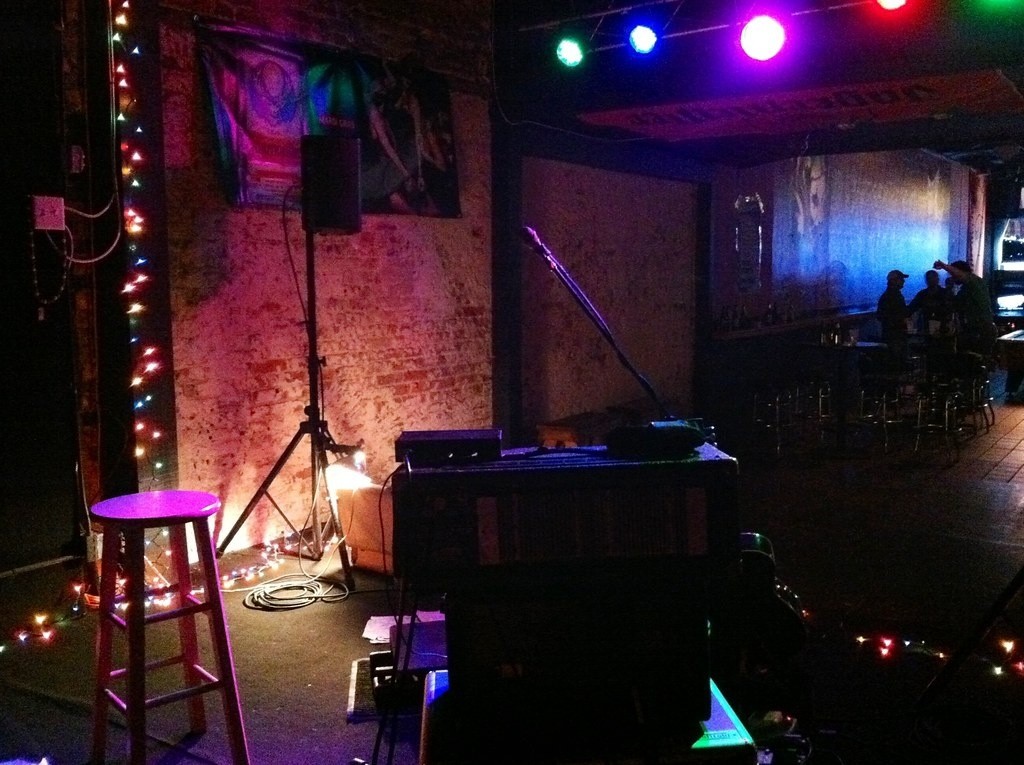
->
[887,271,909,280]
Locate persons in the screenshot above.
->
[877,258,997,388]
[369,86,445,215]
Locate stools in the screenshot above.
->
[535,396,663,449]
[753,343,988,459]
[90,490,250,765]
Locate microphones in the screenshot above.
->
[519,227,557,273]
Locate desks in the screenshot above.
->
[903,331,959,383]
[812,340,887,449]
[372,442,742,764]
[418,670,758,765]
[997,329,1024,396]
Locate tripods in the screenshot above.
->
[214,232,355,592]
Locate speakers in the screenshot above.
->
[442,552,712,729]
[302,135,361,233]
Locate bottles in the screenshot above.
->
[720,287,805,330]
[905,308,960,335]
[819,317,860,346]
